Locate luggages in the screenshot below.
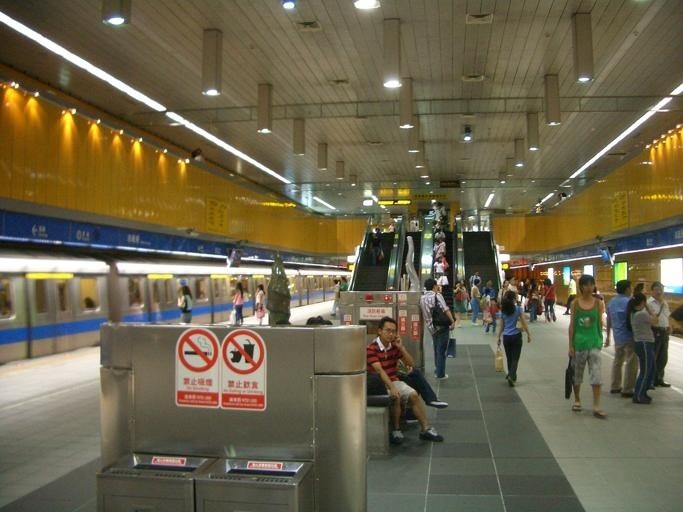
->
[229,309,238,325]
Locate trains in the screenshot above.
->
[0,248,353,367]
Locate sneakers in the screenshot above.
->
[433,373,448,381]
[610,382,671,403]
[388,401,448,445]
[505,375,517,387]
[458,322,497,336]
[529,313,557,323]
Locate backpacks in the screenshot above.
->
[480,295,490,309]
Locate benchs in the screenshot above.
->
[366,407,391,458]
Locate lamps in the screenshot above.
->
[408,115,421,152]
[336,161,345,180]
[463,136,472,141]
[383,18,403,88]
[353,1,382,9]
[350,175,358,186]
[499,171,506,184]
[281,1,294,8]
[424,173,431,185]
[572,13,593,82]
[415,141,425,167]
[526,112,540,151]
[400,78,413,129]
[420,160,429,178]
[506,159,515,177]
[514,138,525,167]
[100,1,131,26]
[0,11,337,209]
[544,74,561,126]
[536,82,683,208]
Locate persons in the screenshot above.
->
[602,280,639,397]
[567,275,607,419]
[388,222,394,238]
[82,296,95,308]
[646,280,671,390]
[374,227,381,234]
[625,293,659,404]
[366,315,444,445]
[329,280,341,317]
[339,277,348,291]
[368,232,382,266]
[231,282,244,325]
[419,278,454,379]
[561,275,576,315]
[427,201,450,287]
[632,277,650,296]
[254,284,266,325]
[176,285,193,325]
[497,291,531,387]
[453,270,556,332]
[397,366,448,424]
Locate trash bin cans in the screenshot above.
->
[410,220,419,232]
[96,452,314,512]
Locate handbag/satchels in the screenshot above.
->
[378,250,384,260]
[467,302,472,311]
[435,275,449,285]
[255,308,266,319]
[494,349,505,372]
[482,310,493,323]
[180,299,187,312]
[461,292,470,300]
[432,305,456,327]
[564,358,573,399]
[537,304,543,315]
[446,336,457,359]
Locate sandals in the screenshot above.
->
[571,403,608,419]
